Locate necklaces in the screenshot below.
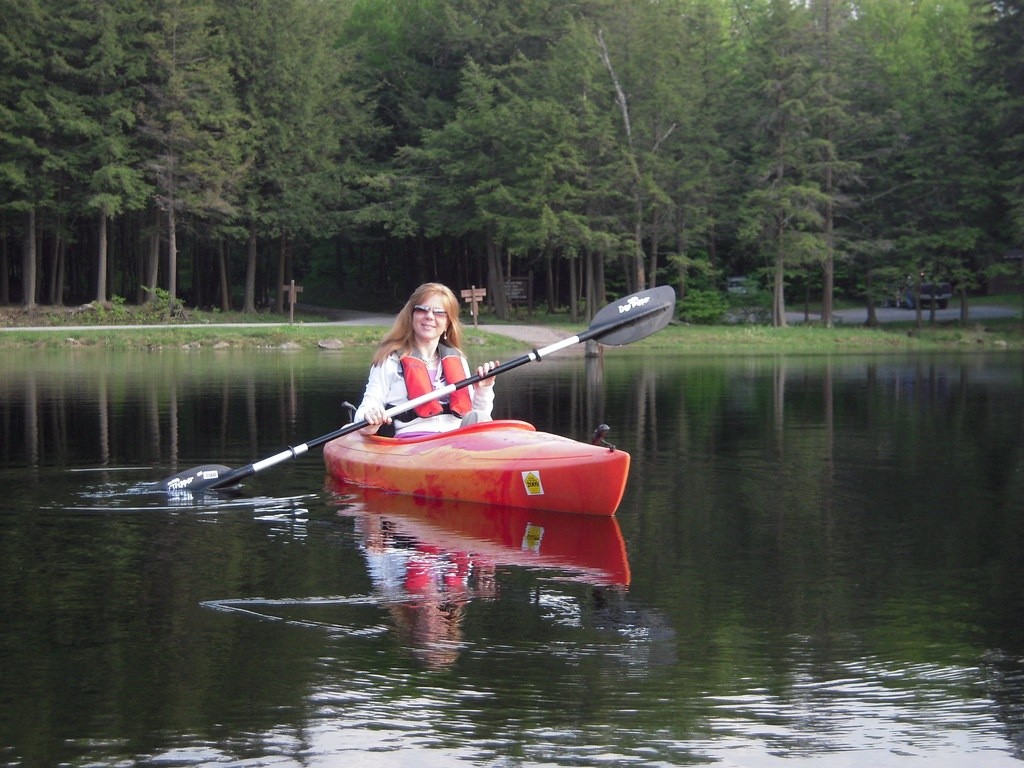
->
[424,354,440,363]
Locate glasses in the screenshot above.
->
[413,304,448,318]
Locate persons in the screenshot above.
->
[354,282,500,438]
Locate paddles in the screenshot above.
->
[153,284,677,494]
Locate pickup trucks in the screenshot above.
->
[889,271,953,308]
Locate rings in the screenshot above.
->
[376,411,379,415]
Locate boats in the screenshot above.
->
[320,474,635,596]
[323,419,631,517]
